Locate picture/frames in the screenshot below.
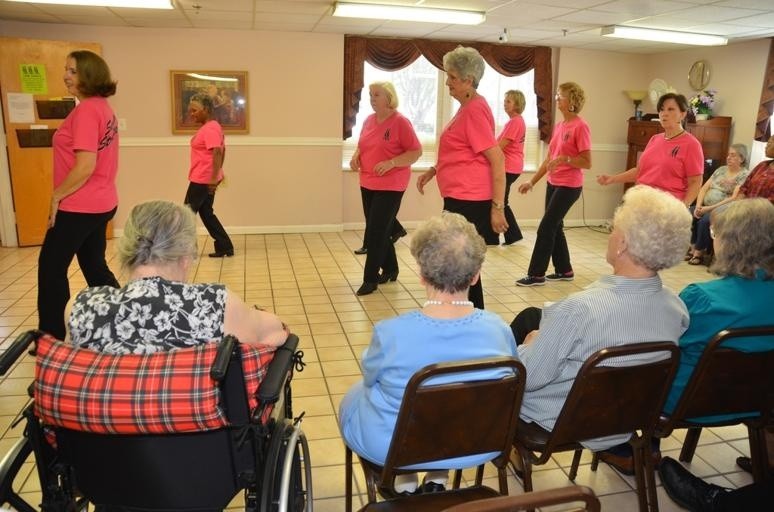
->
[172,70,252,136]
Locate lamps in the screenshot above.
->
[622,88,649,121]
[600,25,732,51]
[326,2,486,28]
[25,1,178,11]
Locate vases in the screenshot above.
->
[695,114,707,123]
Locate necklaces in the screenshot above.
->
[668,128,686,140]
[422,299,478,309]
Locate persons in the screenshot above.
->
[511,183,692,480]
[658,456,773,512]
[183,92,234,258]
[516,81,592,286]
[355,216,407,254]
[27,49,120,356]
[596,93,705,209]
[598,198,774,476]
[485,90,525,246]
[684,143,749,264]
[415,46,508,310]
[335,210,519,497]
[735,134,774,205]
[62,200,292,355]
[204,85,226,111]
[347,81,423,296]
[220,87,233,119]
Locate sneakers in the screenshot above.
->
[544,271,574,281]
[486,241,500,247]
[502,236,523,247]
[515,276,546,286]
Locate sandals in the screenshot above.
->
[684,252,704,265]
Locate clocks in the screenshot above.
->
[685,59,712,90]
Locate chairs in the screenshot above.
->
[655,321,771,511]
[349,484,600,510]
[342,349,524,499]
[513,332,680,511]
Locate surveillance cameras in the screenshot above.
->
[497,33,510,43]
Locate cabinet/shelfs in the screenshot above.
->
[622,114,734,195]
[1,40,116,244]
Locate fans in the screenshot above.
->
[649,77,675,120]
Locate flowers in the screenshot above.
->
[684,87,718,114]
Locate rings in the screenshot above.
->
[500,224,505,229]
[380,169,383,172]
[48,216,52,219]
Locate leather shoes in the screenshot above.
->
[656,456,728,512]
[377,474,446,499]
[703,250,713,266]
[599,451,635,476]
[508,446,524,479]
[643,451,662,470]
[354,246,368,254]
[393,229,407,243]
[736,456,753,473]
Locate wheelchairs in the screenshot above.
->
[1,323,316,512]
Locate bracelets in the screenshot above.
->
[390,158,399,168]
[430,166,438,175]
[566,154,571,165]
[492,200,505,209]
[527,180,533,189]
[683,199,691,207]
[211,175,217,179]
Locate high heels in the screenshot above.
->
[209,247,234,257]
[380,270,399,284]
[357,273,380,296]
